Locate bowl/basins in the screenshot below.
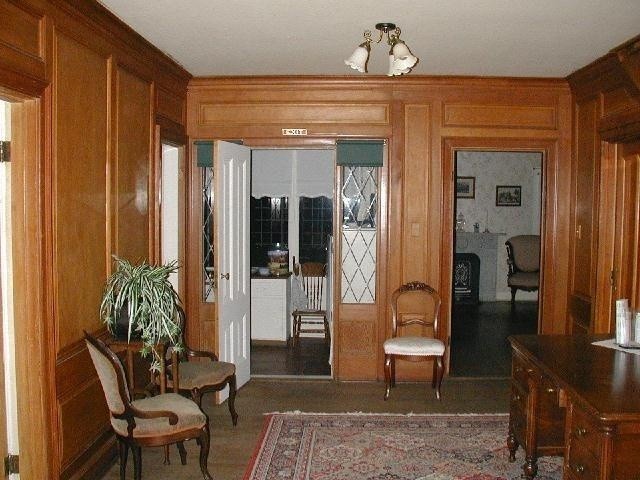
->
[251,266,272,277]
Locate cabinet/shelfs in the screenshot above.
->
[565,395,640,480]
[508,348,564,479]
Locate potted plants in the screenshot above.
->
[99,255,188,376]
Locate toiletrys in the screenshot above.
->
[620,310,633,349]
[615,298,629,345]
[634,312,640,345]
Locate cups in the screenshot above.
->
[615,298,640,349]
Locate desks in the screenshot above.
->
[507,334,640,479]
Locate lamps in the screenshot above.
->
[343,23,418,77]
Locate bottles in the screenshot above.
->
[455,210,468,233]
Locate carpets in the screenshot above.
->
[242,412,564,479]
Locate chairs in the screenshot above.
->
[383,281,445,402]
[81,304,239,480]
[505,235,540,304]
[292,256,330,347]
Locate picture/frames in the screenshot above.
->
[457,176,475,199]
[496,185,521,206]
